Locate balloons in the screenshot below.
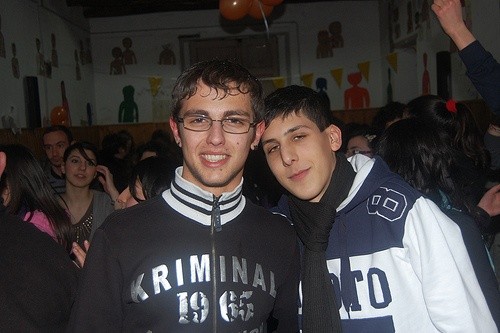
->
[48,106,70,126]
[219,0,281,19]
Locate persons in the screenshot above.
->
[344,0,500,281]
[66,57,299,333]
[260,86,500,333]
[0,124,183,333]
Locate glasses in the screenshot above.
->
[173,113,258,135]
[344,150,373,159]
